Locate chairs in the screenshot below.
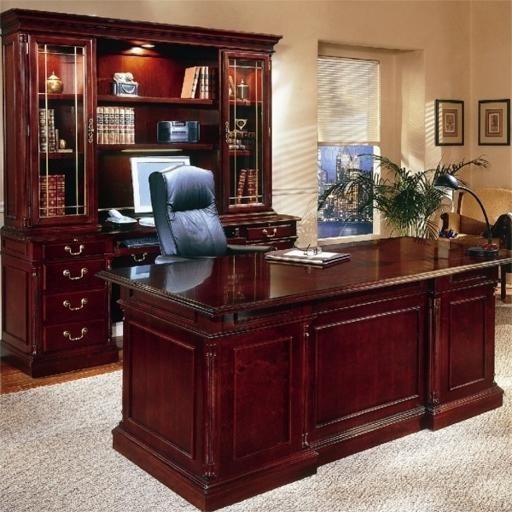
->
[440,187,512,299]
[148,163,273,262]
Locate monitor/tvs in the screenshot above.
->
[129,155,191,227]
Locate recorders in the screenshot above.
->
[156,118,201,144]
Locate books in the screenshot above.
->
[37,108,58,153]
[234,168,256,204]
[40,174,65,216]
[96,106,136,145]
[180,66,209,99]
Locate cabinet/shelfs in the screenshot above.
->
[94,234,512,510]
[0,8,283,377]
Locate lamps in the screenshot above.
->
[434,173,499,257]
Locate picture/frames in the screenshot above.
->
[435,99,465,146]
[478,98,511,145]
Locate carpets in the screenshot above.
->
[0,280,512,511]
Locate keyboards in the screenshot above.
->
[122,236,159,247]
